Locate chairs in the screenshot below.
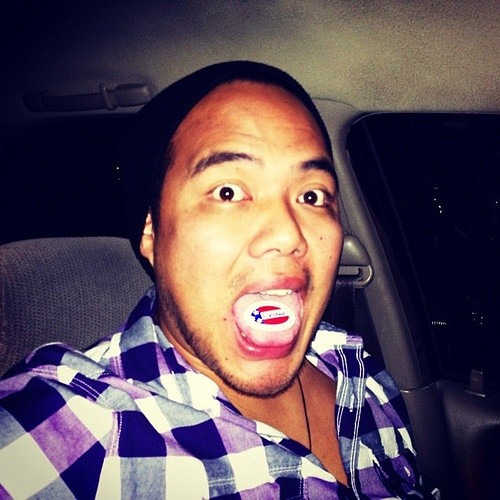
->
[0,236,156,378]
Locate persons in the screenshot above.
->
[0,59,447,500]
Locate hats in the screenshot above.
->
[123,61,334,283]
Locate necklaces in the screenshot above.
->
[295,374,312,451]
